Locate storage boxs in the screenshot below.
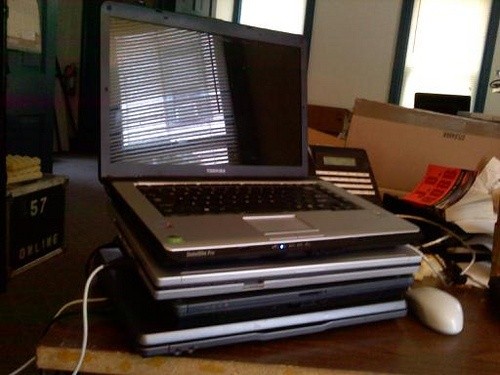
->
[4,174,68,277]
[308,97,500,204]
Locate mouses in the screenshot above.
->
[405,285,464,336]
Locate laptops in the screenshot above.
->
[99,1,422,262]
[173,275,415,319]
[95,242,409,359]
[104,197,422,302]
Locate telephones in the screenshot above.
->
[308,145,381,204]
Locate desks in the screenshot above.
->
[35,155,500,374]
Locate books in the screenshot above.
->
[383,164,491,221]
[309,145,376,196]
